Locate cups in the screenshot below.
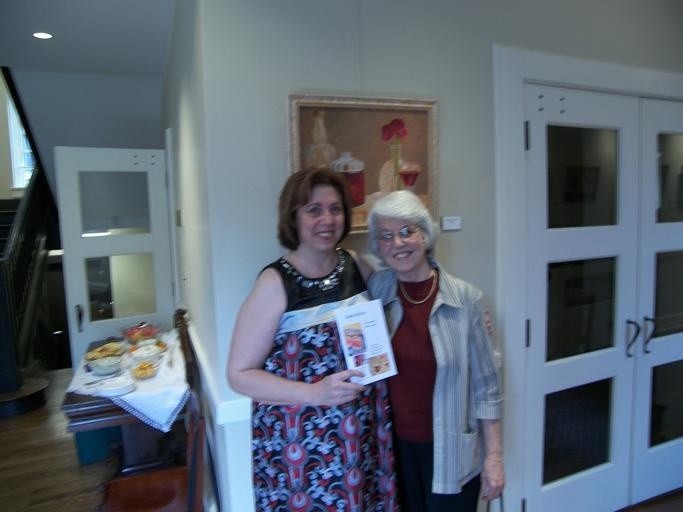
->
[399,161,422,189]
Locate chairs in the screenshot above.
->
[101,392,203,511]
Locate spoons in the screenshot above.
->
[83,370,126,385]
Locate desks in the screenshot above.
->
[59,330,204,478]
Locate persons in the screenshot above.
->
[225,166,495,511]
[365,190,504,511]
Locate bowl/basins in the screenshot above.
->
[87,356,122,376]
[130,361,160,380]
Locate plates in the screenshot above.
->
[94,377,137,399]
[127,338,169,361]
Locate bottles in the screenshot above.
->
[305,109,336,174]
[332,152,365,208]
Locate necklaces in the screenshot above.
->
[399,269,437,305]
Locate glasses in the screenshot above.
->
[377,225,421,243]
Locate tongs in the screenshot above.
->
[132,320,153,328]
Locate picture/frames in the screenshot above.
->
[287,93,439,239]
